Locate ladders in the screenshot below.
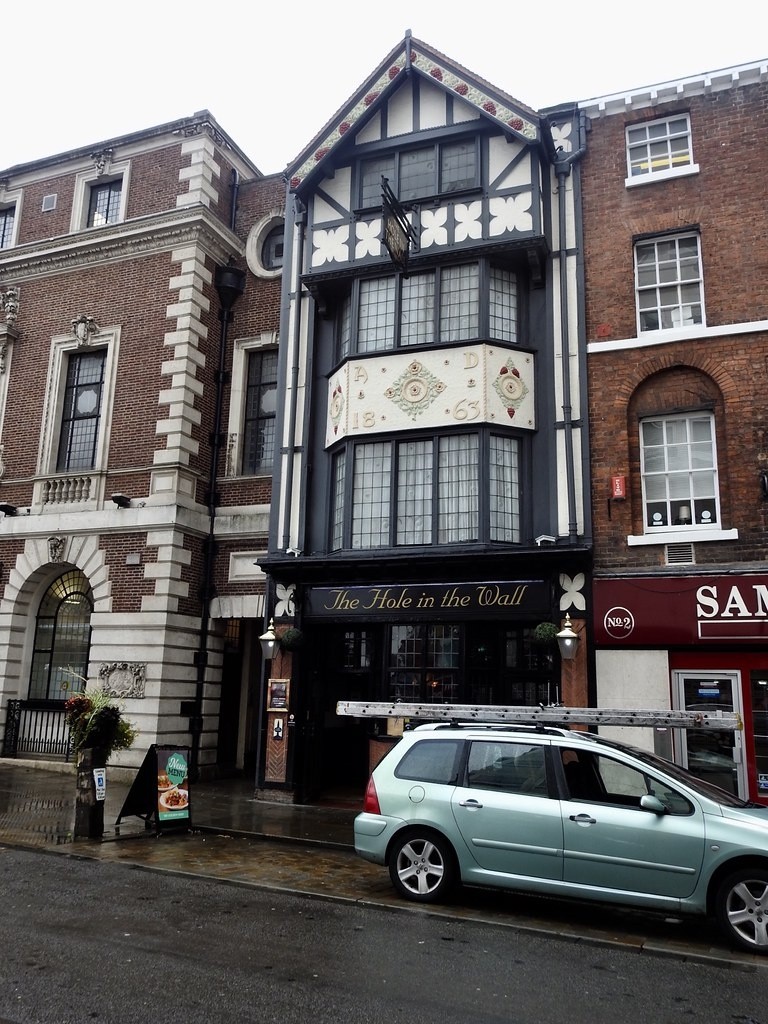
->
[333,697,747,731]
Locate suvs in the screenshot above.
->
[353,720,768,953]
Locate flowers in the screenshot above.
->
[58,666,140,752]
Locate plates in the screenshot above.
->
[160,789,188,809]
[158,776,179,791]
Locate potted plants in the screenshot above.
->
[280,628,304,652]
[535,623,560,654]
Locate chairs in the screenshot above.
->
[567,760,606,801]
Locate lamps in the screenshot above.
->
[111,493,131,509]
[258,618,281,660]
[556,612,581,661]
[0,502,17,516]
